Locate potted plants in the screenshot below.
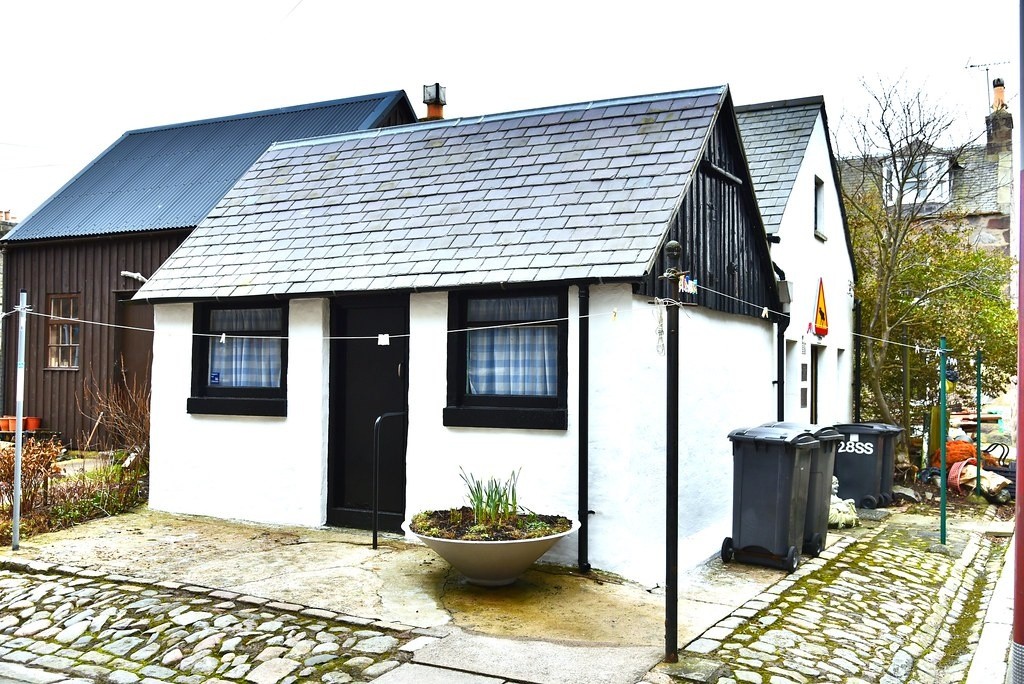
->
[0,415,41,431]
[401,465,581,586]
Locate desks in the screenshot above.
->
[958,421,977,438]
[0,431,36,447]
[963,417,1002,423]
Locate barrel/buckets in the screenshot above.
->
[0,415,41,431]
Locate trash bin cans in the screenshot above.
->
[721,421,845,573]
[833,423,907,508]
